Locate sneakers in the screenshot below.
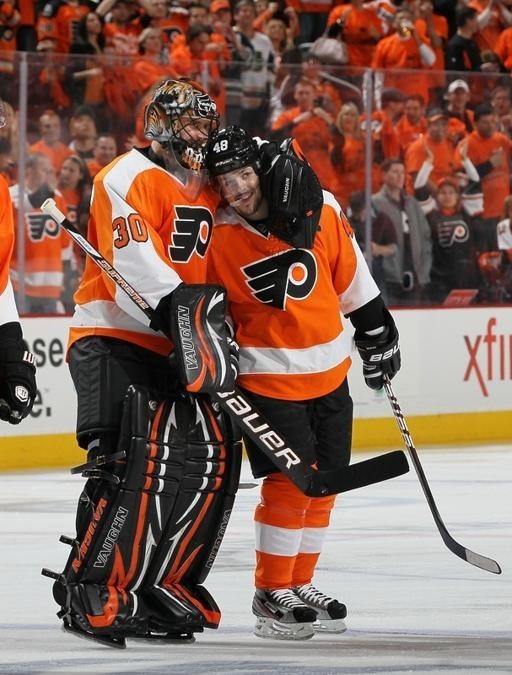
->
[252,588,315,623]
[290,582,347,619]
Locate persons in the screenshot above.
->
[206,123,401,624]
[240,2,510,303]
[0,174,37,425]
[0,1,250,314]
[52,77,323,641]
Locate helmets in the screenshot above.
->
[0,98,16,153]
[135,77,220,202]
[206,124,262,209]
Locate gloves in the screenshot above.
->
[0,336,37,424]
[354,308,400,389]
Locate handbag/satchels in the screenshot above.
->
[308,25,348,65]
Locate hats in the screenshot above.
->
[448,79,470,93]
[427,108,447,122]
[348,192,364,221]
[437,176,458,188]
[74,104,96,119]
[382,89,406,102]
[208,0,230,13]
